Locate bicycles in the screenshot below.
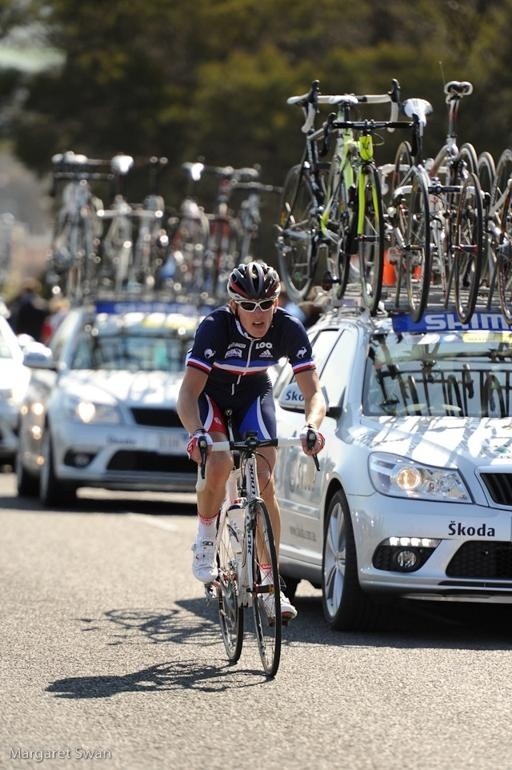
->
[272,74,512,323]
[39,147,279,303]
[194,429,321,681]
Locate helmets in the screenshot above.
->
[227,262,281,304]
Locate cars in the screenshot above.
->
[15,303,223,511]
[269,310,511,631]
[0,308,40,473]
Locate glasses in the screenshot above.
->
[239,299,274,312]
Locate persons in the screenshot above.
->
[276,283,307,322]
[175,259,327,622]
[10,277,61,342]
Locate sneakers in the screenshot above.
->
[265,589,298,620]
[191,533,219,585]
